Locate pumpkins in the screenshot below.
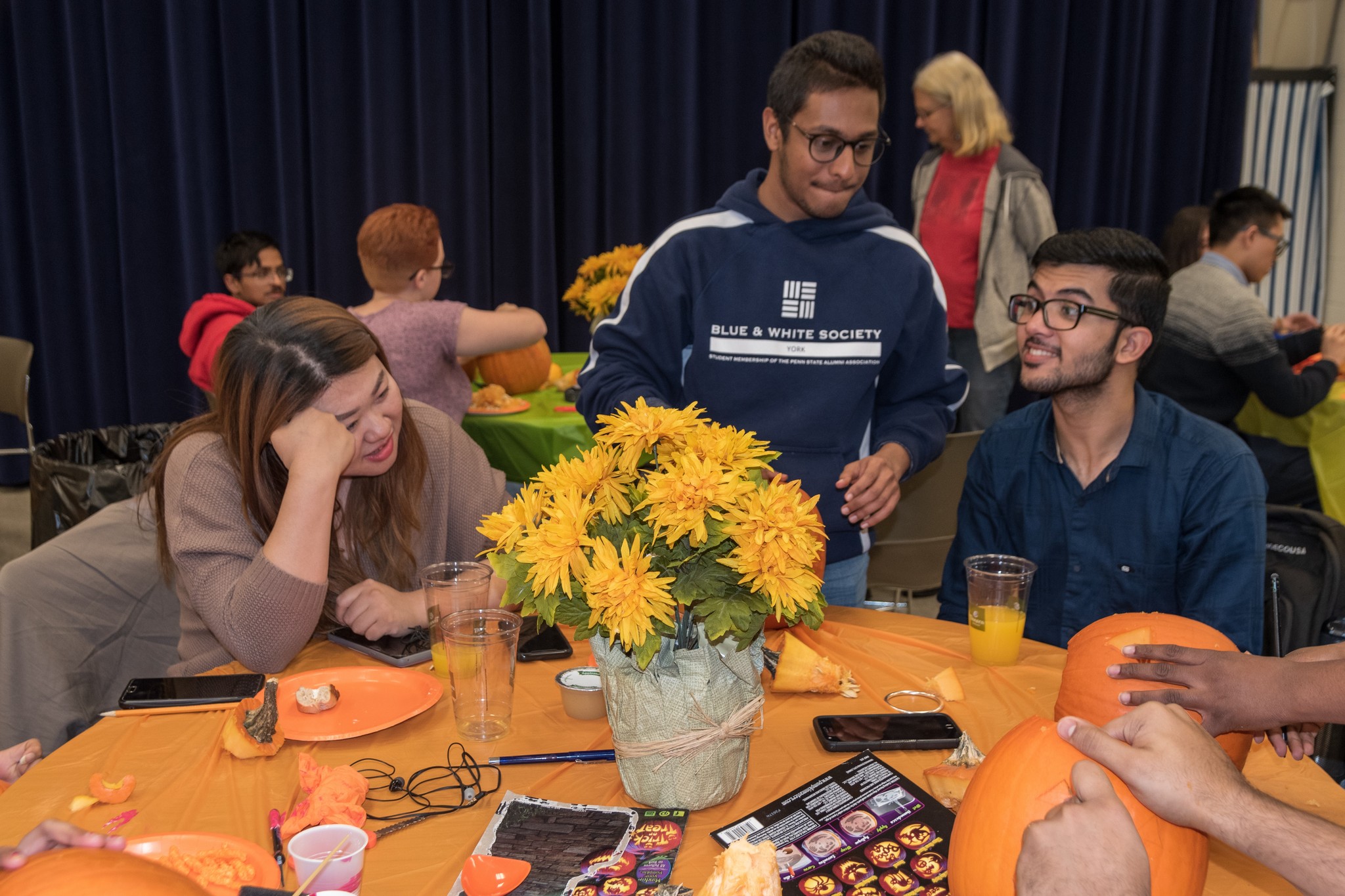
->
[461,335,551,395]
[764,480,826,629]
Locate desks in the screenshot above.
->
[1234,380,1345,526]
[0,606,1345,896]
[463,352,599,485]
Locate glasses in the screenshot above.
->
[918,103,946,119]
[1260,229,1290,256]
[408,261,454,280]
[1009,293,1121,331]
[766,102,891,165]
[243,265,293,281]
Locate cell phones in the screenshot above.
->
[324,625,433,668]
[811,712,963,752]
[499,614,572,663]
[120,673,265,710]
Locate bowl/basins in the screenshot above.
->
[305,890,357,896]
[555,665,607,720]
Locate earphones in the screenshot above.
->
[390,777,405,791]
[464,784,475,801]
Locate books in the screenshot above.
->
[445,788,691,896]
[707,749,950,896]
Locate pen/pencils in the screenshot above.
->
[489,749,616,766]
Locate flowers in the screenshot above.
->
[475,397,827,670]
[563,243,646,318]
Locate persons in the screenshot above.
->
[1011,699,1345,896]
[577,29,958,610]
[1139,184,1345,455]
[902,52,1056,439]
[937,228,1267,667]
[0,737,43,783]
[344,202,550,436]
[138,295,523,675]
[1106,634,1345,763]
[1161,205,1317,336]
[177,231,296,396]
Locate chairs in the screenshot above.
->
[1265,504,1345,783]
[866,433,985,619]
[0,337,35,456]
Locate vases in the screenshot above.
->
[588,620,762,810]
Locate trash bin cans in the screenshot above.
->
[30,424,181,550]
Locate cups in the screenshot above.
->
[963,553,1039,666]
[416,560,495,679]
[438,608,524,743]
[288,823,369,896]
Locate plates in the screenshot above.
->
[124,831,281,896]
[254,665,444,741]
[467,398,531,415]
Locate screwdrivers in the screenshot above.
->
[270,809,286,887]
